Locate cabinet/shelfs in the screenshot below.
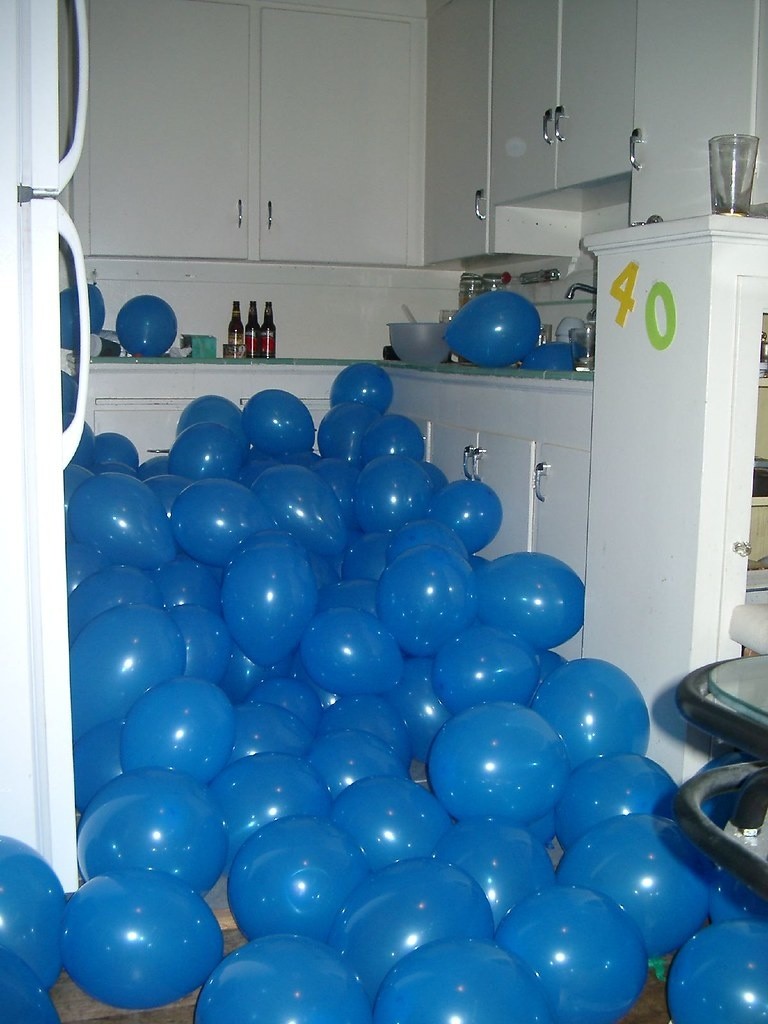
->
[71,0,768,268]
[87,364,595,665]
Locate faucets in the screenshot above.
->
[565,283,597,299]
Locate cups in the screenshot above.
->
[483,274,504,293]
[537,324,552,346]
[439,310,459,323]
[570,328,596,372]
[710,134,759,218]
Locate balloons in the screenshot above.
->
[329,362,393,417]
[61,368,768,1024]
[60,284,106,350]
[1,834,67,991]
[442,290,541,368]
[0,946,62,1024]
[519,341,587,371]
[116,294,179,358]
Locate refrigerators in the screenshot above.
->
[0,0,89,885]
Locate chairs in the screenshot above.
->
[674,652,768,897]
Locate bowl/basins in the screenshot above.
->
[386,323,450,363]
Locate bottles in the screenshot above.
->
[228,301,245,344]
[261,301,276,359]
[245,301,261,359]
[458,272,482,310]
